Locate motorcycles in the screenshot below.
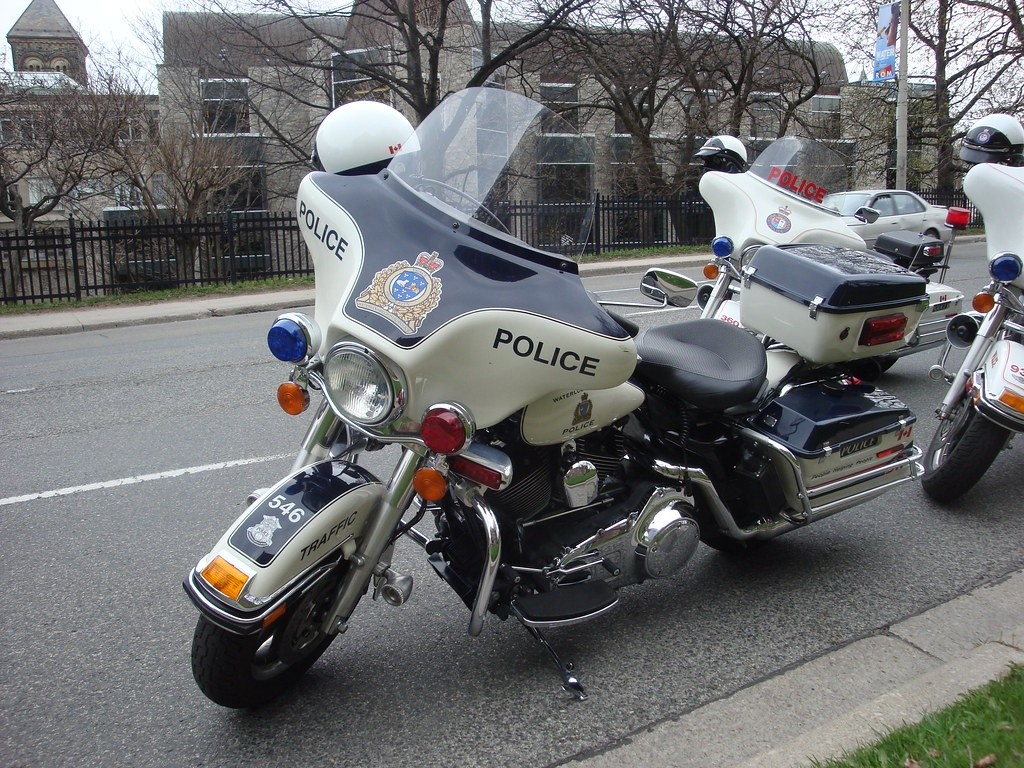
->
[182,87,929,710]
[639,135,970,373]
[921,164,1024,503]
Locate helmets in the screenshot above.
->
[316,99,422,174]
[960,114,1024,163]
[695,134,748,173]
